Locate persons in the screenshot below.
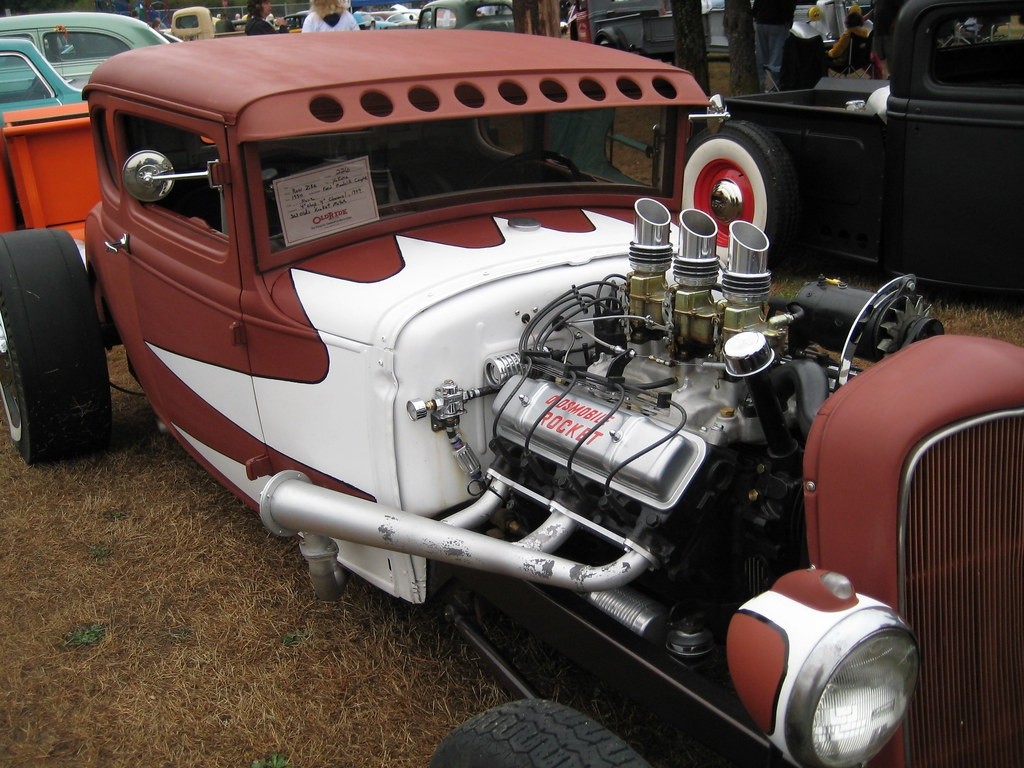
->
[302,0,360,32]
[751,0,796,85]
[245,0,289,35]
[132,11,138,18]
[235,14,242,30]
[826,12,868,73]
[152,17,167,32]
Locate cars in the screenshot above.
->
[384,11,420,30]
[563,0,709,66]
[281,10,311,33]
[363,3,408,21]
[696,0,875,59]
[0,11,172,93]
[0,36,89,129]
[232,20,248,33]
[709,0,1023,312]
[0,22,1023,767]
[415,1,515,31]
[159,8,246,40]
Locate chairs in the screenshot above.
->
[828,30,875,78]
[389,139,487,198]
[763,33,824,93]
[937,17,1012,47]
